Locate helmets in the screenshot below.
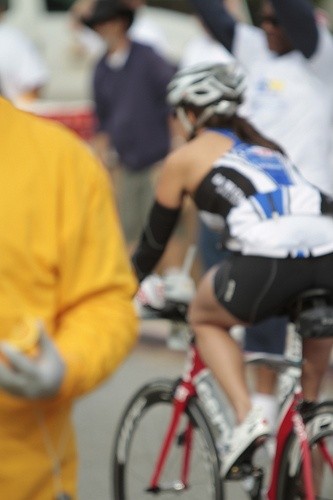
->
[168,55,245,105]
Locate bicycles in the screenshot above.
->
[111,290,333,500]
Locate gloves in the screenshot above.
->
[0,323,65,401]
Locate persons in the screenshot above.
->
[0,0,333,216]
[1,97,139,500]
[131,63,333,482]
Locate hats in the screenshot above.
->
[81,0,135,31]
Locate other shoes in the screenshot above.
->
[218,410,271,480]
